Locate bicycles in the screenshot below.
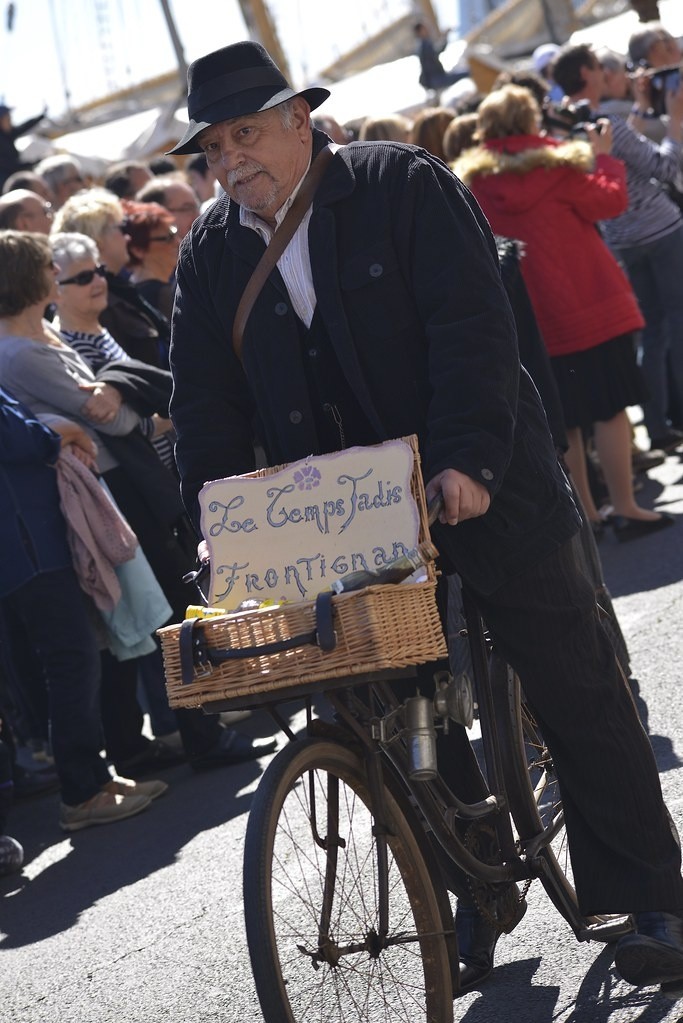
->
[180,489,643,1023]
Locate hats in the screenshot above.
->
[165,42,330,155]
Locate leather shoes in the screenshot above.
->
[452,881,527,993]
[615,911,683,1000]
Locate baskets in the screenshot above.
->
[156,434,448,708]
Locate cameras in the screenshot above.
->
[569,122,602,141]
[540,99,594,130]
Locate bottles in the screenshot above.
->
[307,540,439,601]
[185,597,302,620]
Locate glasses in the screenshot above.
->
[59,265,105,285]
[149,225,178,243]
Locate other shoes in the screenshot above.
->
[12,773,60,804]
[189,729,278,774]
[0,835,24,877]
[100,775,169,799]
[651,429,683,449]
[58,790,152,833]
[611,511,675,541]
[632,449,666,472]
[590,518,603,543]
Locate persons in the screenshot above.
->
[171,40,683,994]
[0,22,683,829]
